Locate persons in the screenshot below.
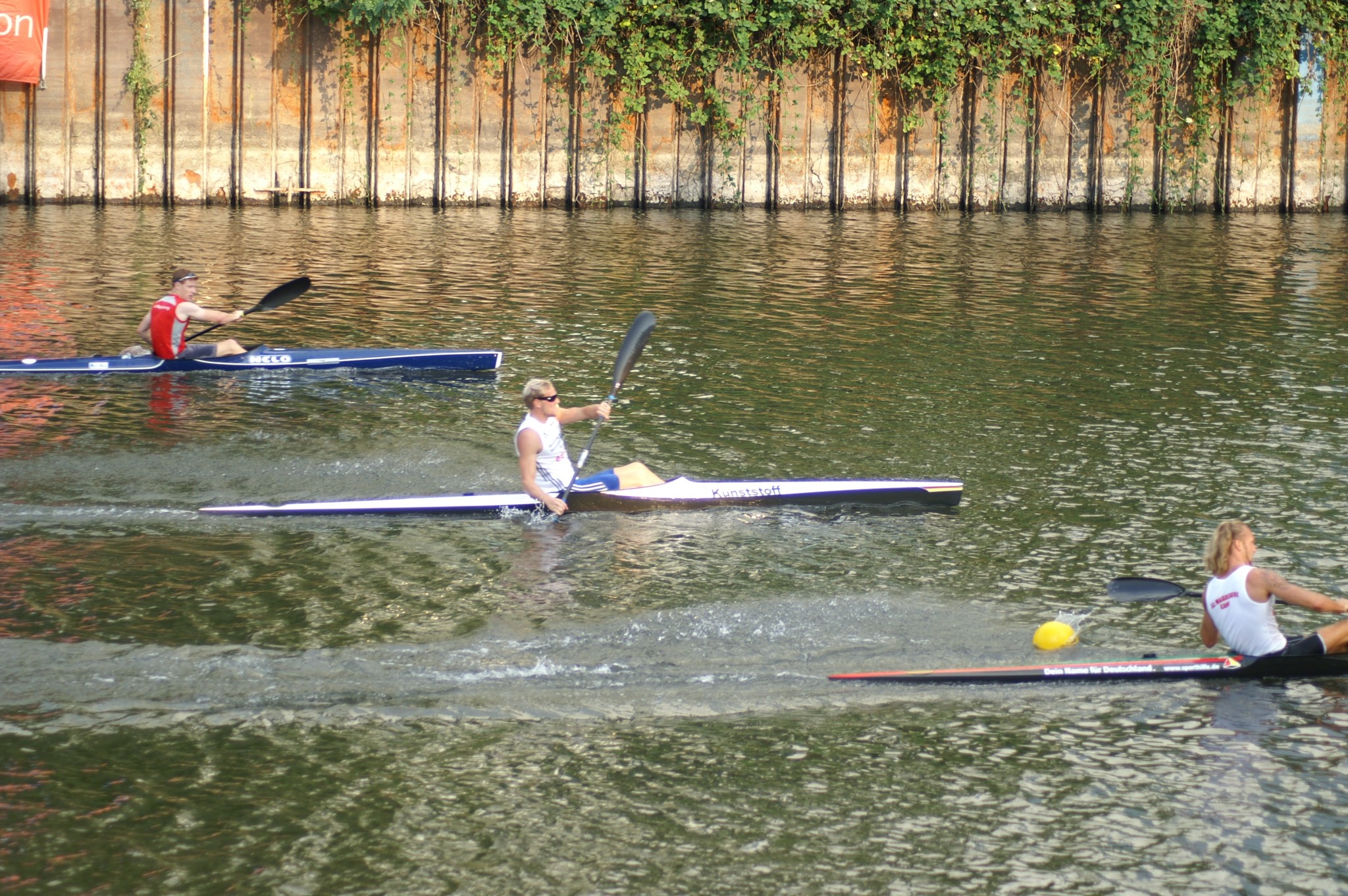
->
[135,268,248,361]
[515,378,665,516]
[1199,518,1347,660]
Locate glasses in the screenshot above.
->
[178,272,199,282]
[534,394,558,402]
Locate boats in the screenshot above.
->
[197,475,964,518]
[0,345,505,374]
[827,651,1348,683]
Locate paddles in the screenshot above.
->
[550,311,656,523]
[1105,575,1289,606]
[144,277,311,357]
